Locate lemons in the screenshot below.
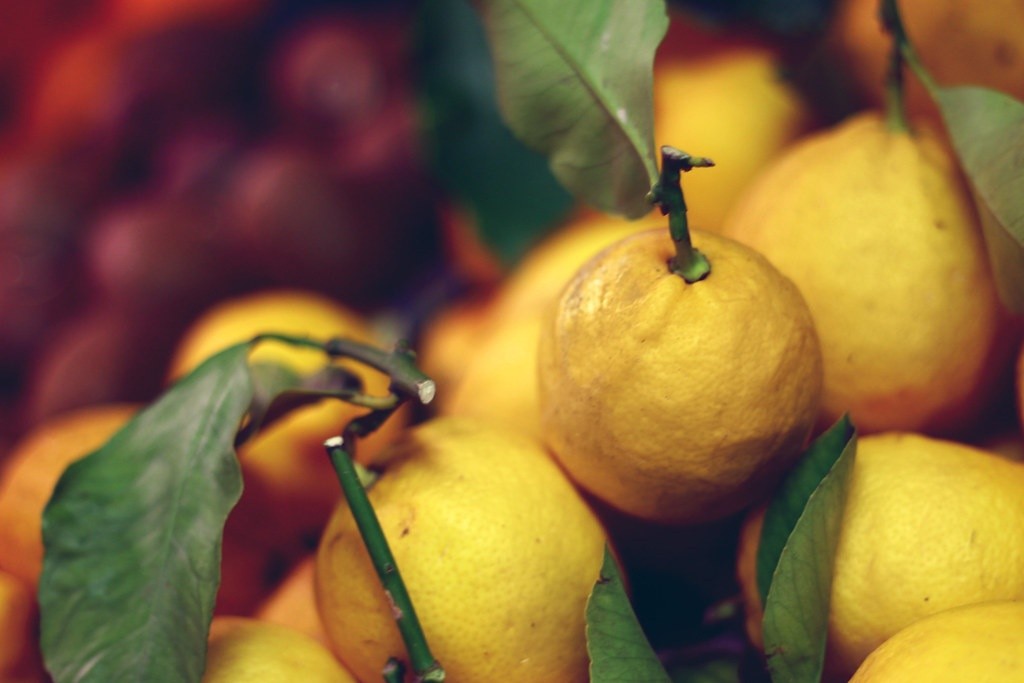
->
[0,0,1024,683]
